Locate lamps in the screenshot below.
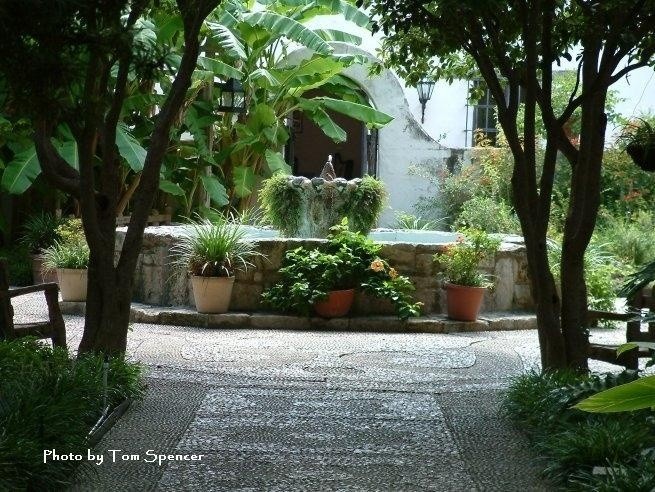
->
[414,72,436,123]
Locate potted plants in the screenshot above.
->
[251,215,431,323]
[36,219,89,300]
[619,63,655,175]
[430,220,508,323]
[162,204,267,316]
